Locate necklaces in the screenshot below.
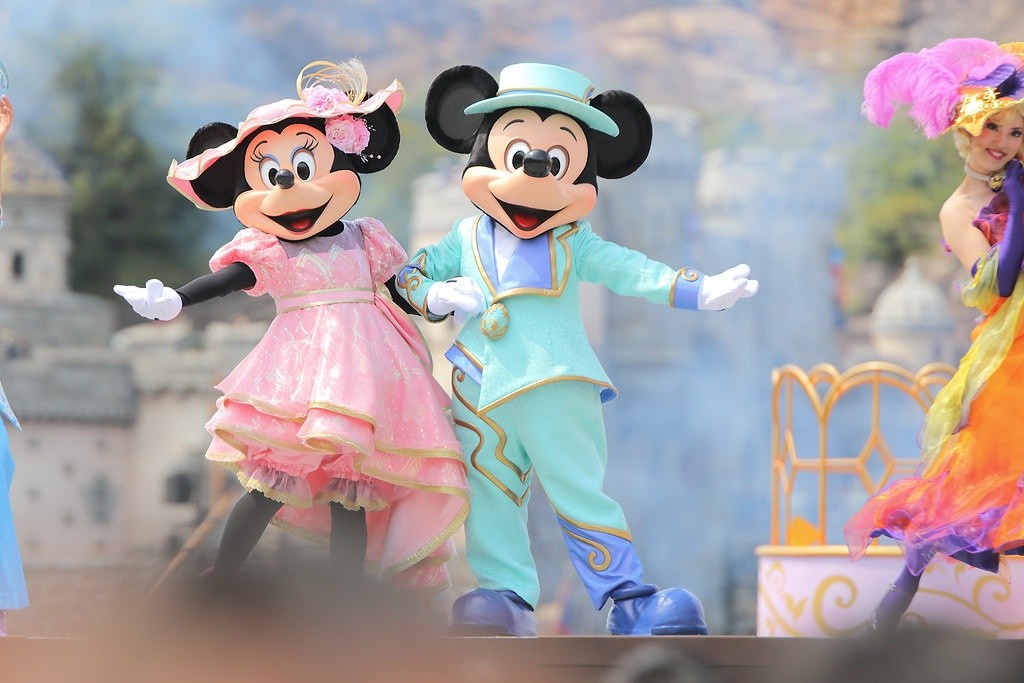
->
[963,164,1008,193]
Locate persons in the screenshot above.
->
[0,94,31,638]
[843,38,1024,634]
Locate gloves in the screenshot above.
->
[971,160,1024,297]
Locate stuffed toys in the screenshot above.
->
[114,58,450,629]
[396,63,760,638]
